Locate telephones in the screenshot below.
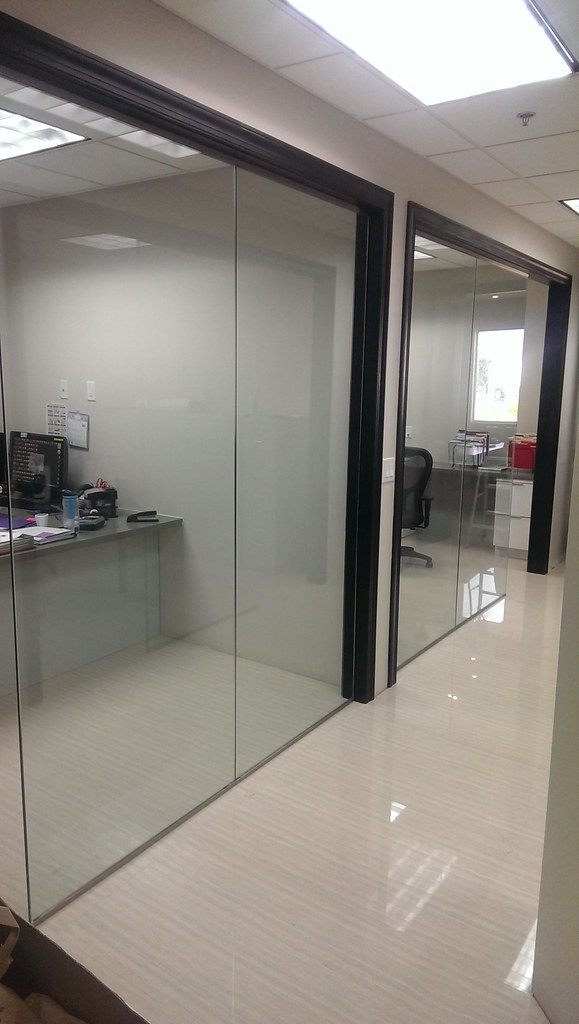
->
[72,484,93,509]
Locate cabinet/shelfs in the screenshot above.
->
[494,479,534,559]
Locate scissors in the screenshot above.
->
[95,478,108,488]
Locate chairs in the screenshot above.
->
[402,446,434,568]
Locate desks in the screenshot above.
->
[0,506,183,695]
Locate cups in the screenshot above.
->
[62,493,80,534]
[35,513,49,527]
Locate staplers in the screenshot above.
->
[126,511,159,522]
